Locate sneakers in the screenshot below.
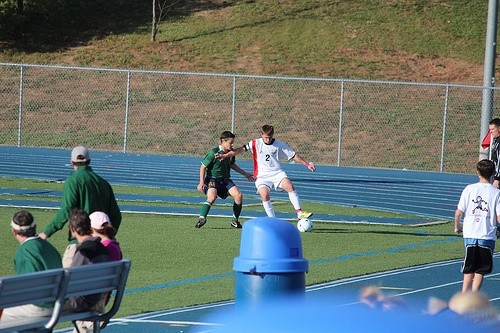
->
[298,210,313,219]
[195,217,207,227]
[230,220,242,228]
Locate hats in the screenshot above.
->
[71,145,90,162]
[89,210,112,230]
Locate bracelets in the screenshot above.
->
[305,163,310,167]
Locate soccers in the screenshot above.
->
[297,218,313,232]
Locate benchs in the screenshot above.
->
[0,258,132,333]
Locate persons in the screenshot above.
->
[195,131,254,228]
[36,145,122,246]
[0,210,63,333]
[214,125,316,220]
[488,117,500,189]
[58,208,110,315]
[454,159,500,295]
[73,210,122,333]
